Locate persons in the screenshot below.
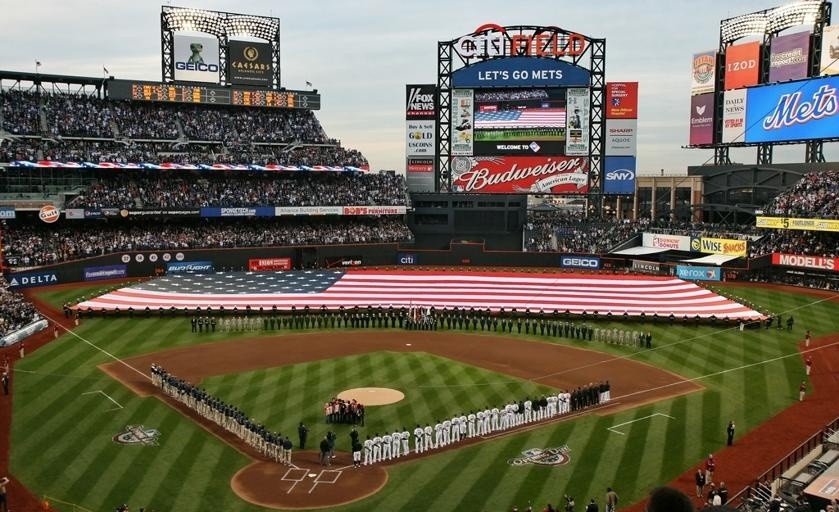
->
[0,87,838,512]
